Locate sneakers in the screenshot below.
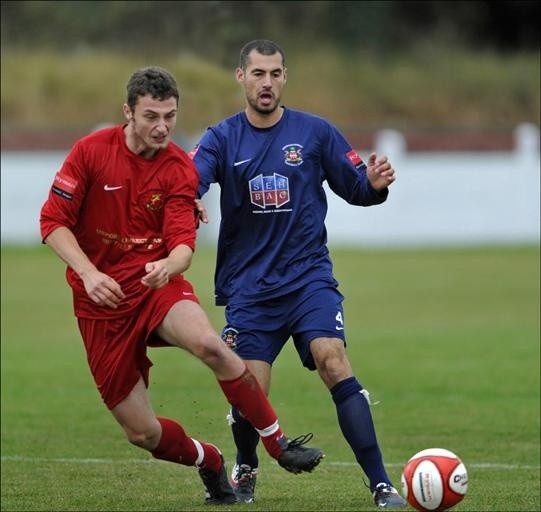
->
[366,482,407,509]
[198,441,235,505]
[232,462,258,504]
[276,439,324,474]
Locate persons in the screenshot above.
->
[184,38,412,508]
[38,64,324,506]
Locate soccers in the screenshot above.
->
[401,448,468,512]
